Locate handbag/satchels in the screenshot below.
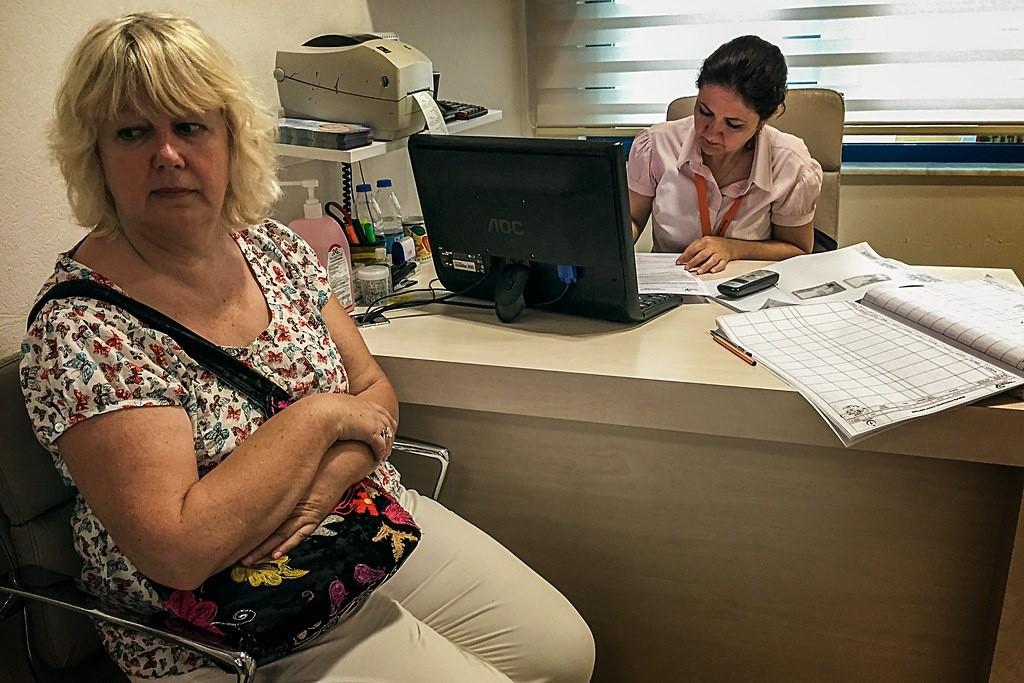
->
[146,396,421,673]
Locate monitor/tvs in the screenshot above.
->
[407,133,644,326]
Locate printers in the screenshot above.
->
[273,32,434,141]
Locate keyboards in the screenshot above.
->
[639,293,683,321]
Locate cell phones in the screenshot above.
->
[717,270,779,298]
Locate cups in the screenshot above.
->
[359,266,389,306]
[403,216,432,262]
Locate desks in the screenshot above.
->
[352,257,1024,683]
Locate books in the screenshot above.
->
[715,275,1024,446]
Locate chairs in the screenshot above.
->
[667,87,846,258]
[0,351,450,683]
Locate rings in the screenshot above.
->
[383,427,392,437]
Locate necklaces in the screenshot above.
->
[719,153,743,181]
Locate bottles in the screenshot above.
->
[374,179,404,254]
[353,183,386,248]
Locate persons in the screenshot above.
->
[20,11,595,683]
[628,35,823,275]
